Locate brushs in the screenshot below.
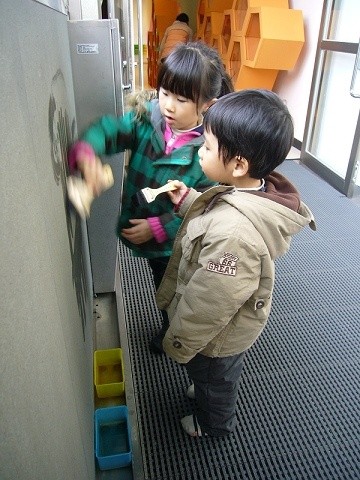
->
[132,179,181,206]
[64,164,114,220]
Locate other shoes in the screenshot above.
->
[179,413,210,439]
[150,335,170,355]
[187,384,194,399]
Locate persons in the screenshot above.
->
[154,88,317,438]
[159,13,194,63]
[68,40,235,354]
